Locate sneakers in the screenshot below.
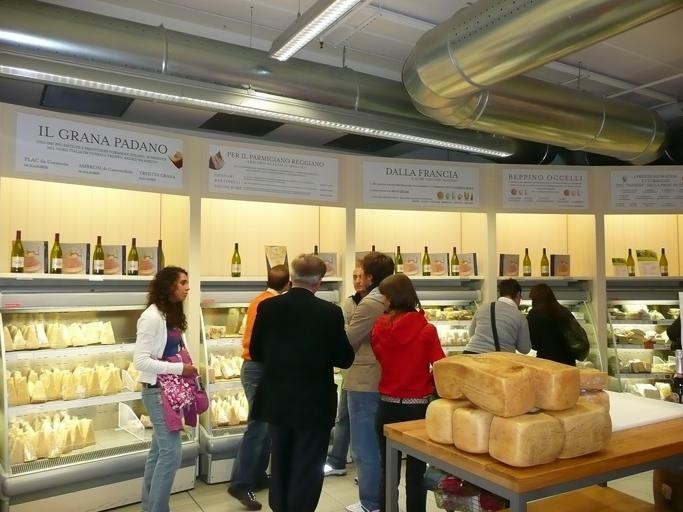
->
[349,504,379,512]
[322,462,347,477]
[251,471,275,491]
[344,500,361,508]
[227,482,262,511]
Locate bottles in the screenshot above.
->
[50,233,62,273]
[422,247,431,276]
[93,236,104,274]
[523,248,531,275]
[11,231,24,272]
[314,245,317,257]
[372,245,375,254]
[231,244,242,277]
[451,247,460,274]
[627,249,635,276]
[659,248,668,276]
[156,240,165,274]
[541,248,548,275]
[394,246,403,273]
[127,238,139,275]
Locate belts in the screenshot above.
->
[380,394,430,405]
[138,382,161,389]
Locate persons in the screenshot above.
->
[250,254,355,512]
[345,253,395,511]
[371,273,447,512]
[461,279,530,355]
[133,265,197,512]
[227,265,291,509]
[323,260,366,483]
[525,283,590,368]
[666,315,683,403]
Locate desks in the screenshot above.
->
[383,389,682,511]
